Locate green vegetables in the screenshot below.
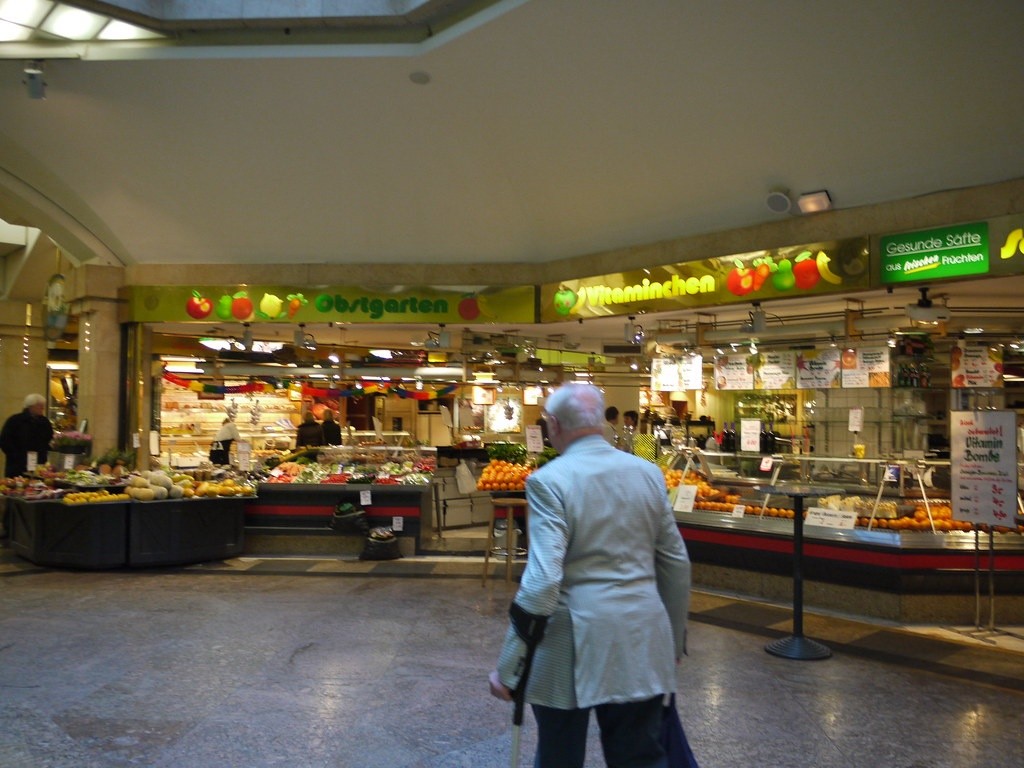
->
[485,441,558,469]
[66,449,134,488]
[293,456,378,486]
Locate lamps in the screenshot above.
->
[797,190,832,213]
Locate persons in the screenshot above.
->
[295,410,325,446]
[488,384,699,768]
[605,406,620,433]
[209,418,240,465]
[321,409,343,445]
[0,393,54,480]
[623,411,640,434]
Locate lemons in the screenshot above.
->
[63,490,131,504]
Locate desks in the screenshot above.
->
[759,483,846,661]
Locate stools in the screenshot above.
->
[481,497,530,584]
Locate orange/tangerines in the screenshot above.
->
[478,458,534,490]
[662,469,1024,534]
